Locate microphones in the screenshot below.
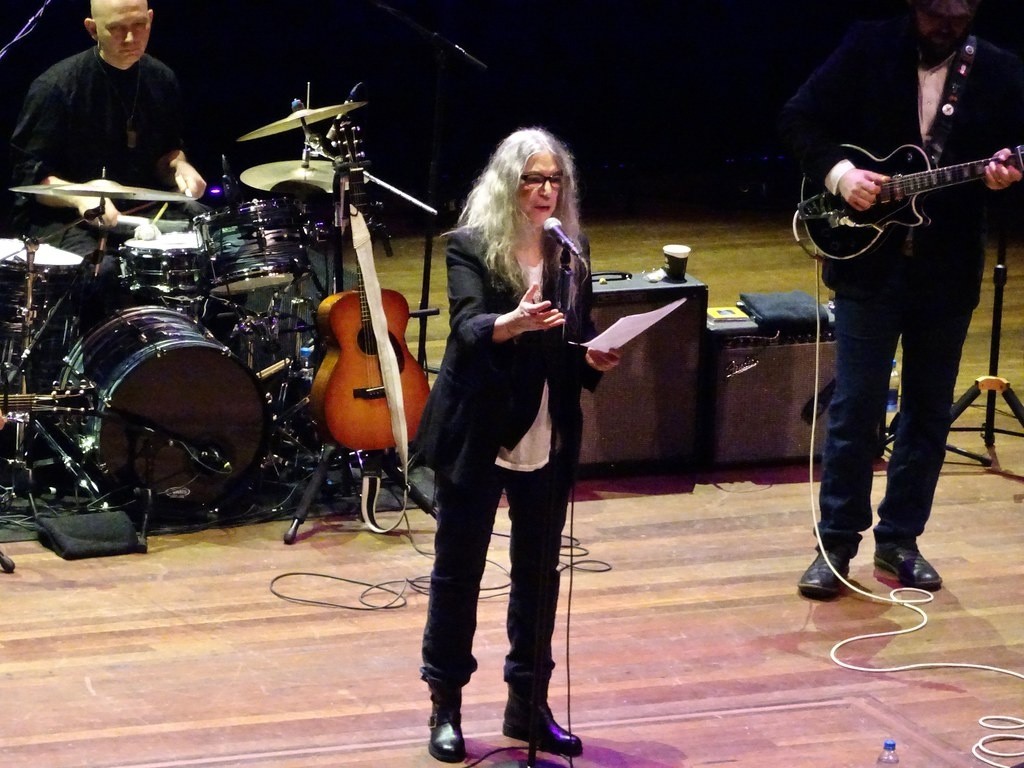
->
[205,444,232,472]
[93,232,109,276]
[801,377,837,425]
[222,155,244,209]
[544,217,580,256]
[325,88,358,140]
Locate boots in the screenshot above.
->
[428,689,465,761]
[503,678,583,754]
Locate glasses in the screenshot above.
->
[519,173,564,189]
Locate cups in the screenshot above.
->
[662,245,691,283]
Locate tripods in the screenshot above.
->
[948,181,1024,432]
[73,408,221,554]
[0,251,101,521]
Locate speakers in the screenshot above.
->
[705,303,837,468]
[570,269,709,475]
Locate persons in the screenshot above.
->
[10,0,249,351]
[415,129,623,763]
[776,0,1024,600]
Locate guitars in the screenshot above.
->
[798,139,1024,261]
[307,118,433,455]
[0,378,98,426]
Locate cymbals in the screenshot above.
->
[7,176,199,202]
[235,101,368,141]
[238,158,372,195]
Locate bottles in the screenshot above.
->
[877,741,900,768]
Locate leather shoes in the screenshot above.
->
[875,542,943,587]
[799,551,850,594]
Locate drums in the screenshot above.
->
[0,237,87,336]
[192,195,314,292]
[124,230,209,301]
[57,302,273,505]
